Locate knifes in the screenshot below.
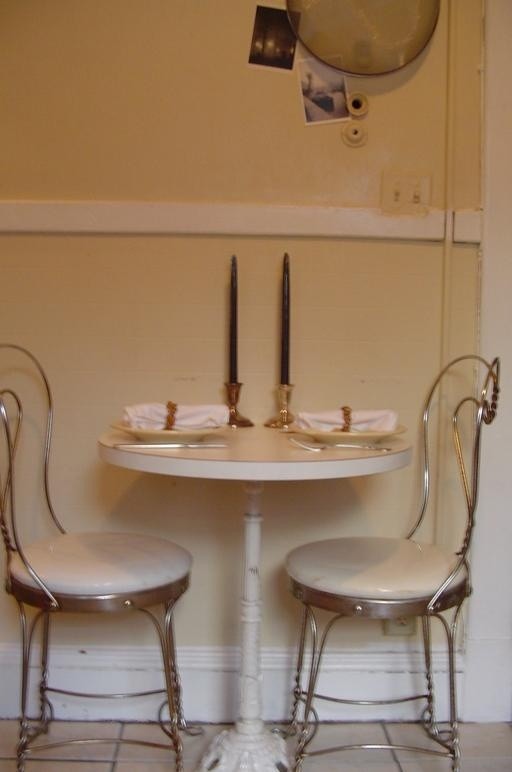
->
[113,442,229,451]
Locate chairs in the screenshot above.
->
[288,351,503,772]
[1,341,207,771]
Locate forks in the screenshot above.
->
[288,436,393,453]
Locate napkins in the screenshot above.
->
[293,406,401,433]
[126,402,231,429]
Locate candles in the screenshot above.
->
[225,250,295,389]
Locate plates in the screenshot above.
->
[279,422,408,442]
[109,420,230,442]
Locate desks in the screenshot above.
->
[96,415,411,771]
